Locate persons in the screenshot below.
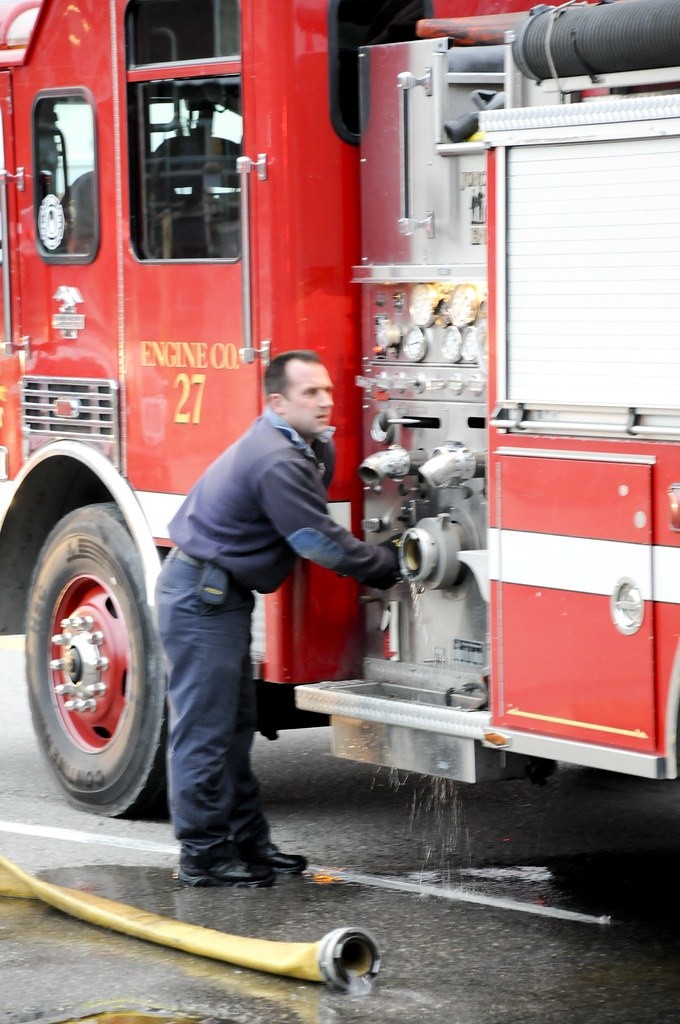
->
[154,351,398,889]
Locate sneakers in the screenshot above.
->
[177,855,275,887]
[247,853,307,875]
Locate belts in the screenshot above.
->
[168,546,207,569]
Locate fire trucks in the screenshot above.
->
[0,0,679,822]
